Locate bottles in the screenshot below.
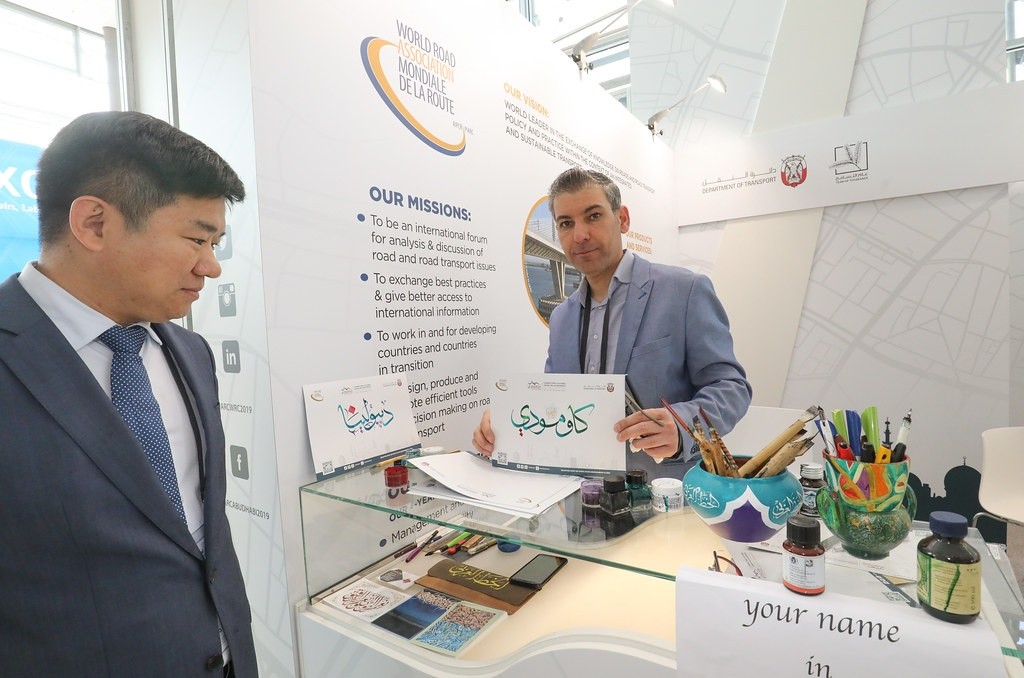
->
[799,463,828,519]
[782,516,826,596]
[917,510,983,625]
[402,448,424,472]
[602,469,653,515]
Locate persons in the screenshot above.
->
[472,165,753,506]
[0,110,259,678]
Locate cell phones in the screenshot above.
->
[509,553,569,591]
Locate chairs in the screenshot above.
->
[971,426,1024,529]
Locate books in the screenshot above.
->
[325,577,508,658]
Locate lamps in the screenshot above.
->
[569,0,678,75]
[647,75,727,136]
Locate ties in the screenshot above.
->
[98,325,188,530]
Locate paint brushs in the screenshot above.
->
[369,454,405,471]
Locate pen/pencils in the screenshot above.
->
[816,406,914,464]
[394,522,503,562]
